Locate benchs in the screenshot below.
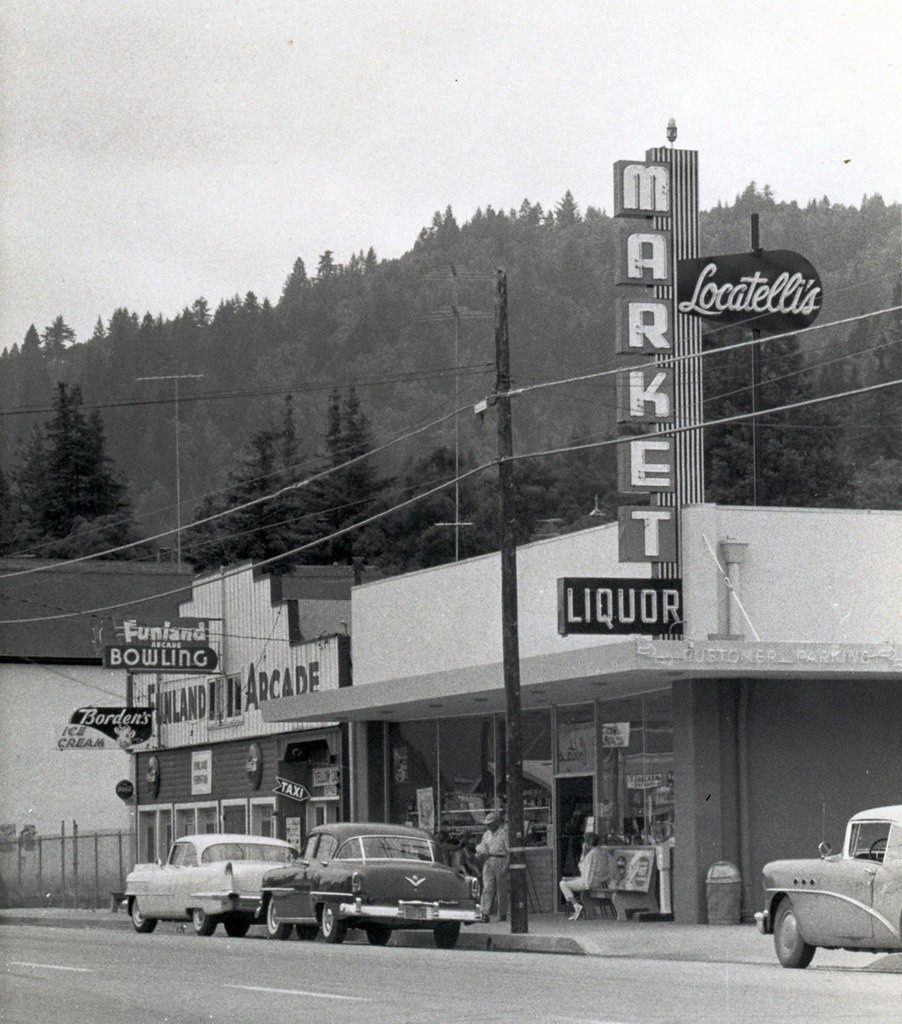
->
[587,845,660,921]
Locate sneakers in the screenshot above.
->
[568,904,583,921]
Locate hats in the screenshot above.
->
[483,813,498,823]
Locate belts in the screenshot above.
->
[489,855,504,857]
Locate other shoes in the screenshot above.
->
[480,917,489,922]
[499,915,506,921]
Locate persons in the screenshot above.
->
[473,812,511,922]
[558,832,612,922]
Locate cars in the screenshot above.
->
[754,805,902,968]
[256,823,482,947]
[122,828,302,937]
